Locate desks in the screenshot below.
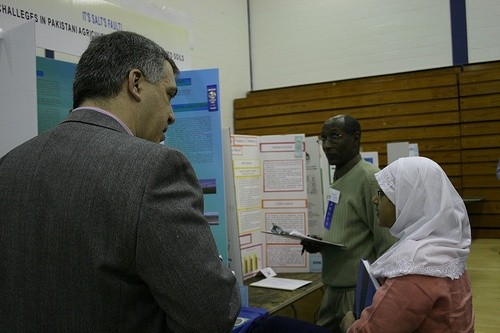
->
[232,272,326,333]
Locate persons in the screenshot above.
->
[341,157,474,333]
[0,32,240,333]
[300,114,400,333]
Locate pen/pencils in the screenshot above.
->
[301,235,310,255]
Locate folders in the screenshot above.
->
[353,259,381,320]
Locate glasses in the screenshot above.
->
[316,133,354,143]
[377,189,383,196]
